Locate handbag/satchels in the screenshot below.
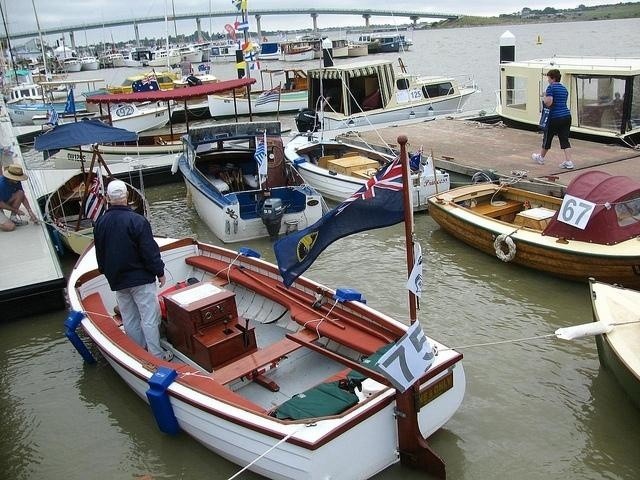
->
[538,100,552,129]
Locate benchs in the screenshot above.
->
[470,197,526,223]
[242,171,261,187]
[81,284,268,416]
[183,254,389,354]
[208,329,324,384]
[209,173,231,193]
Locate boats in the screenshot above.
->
[285,131,448,213]
[425,168,640,291]
[66,135,466,480]
[496,55,640,151]
[588,277,640,412]
[176,121,321,244]
[35,119,152,250]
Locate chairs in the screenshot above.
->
[318,155,336,170]
[341,151,362,158]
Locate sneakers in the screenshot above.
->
[159,350,174,362]
[531,152,545,165]
[558,160,574,169]
[9,214,29,227]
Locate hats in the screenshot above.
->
[2,163,28,182]
[106,179,128,199]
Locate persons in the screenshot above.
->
[611,92,624,119]
[532,69,574,169]
[93,179,174,363]
[0,164,40,232]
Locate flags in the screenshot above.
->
[236,1,261,73]
[254,131,269,184]
[273,152,406,290]
[47,104,58,125]
[132,70,160,93]
[405,242,423,299]
[255,84,281,106]
[62,91,75,118]
[84,163,108,222]
[410,149,421,175]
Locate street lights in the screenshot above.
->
[498,30,517,105]
